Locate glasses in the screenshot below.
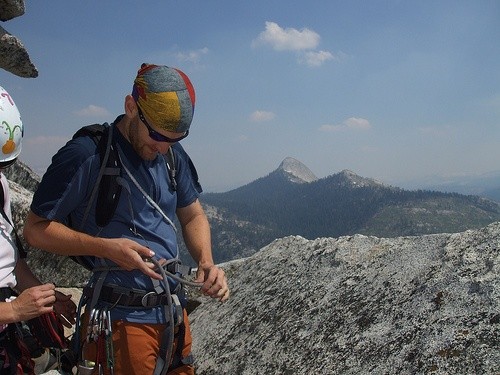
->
[135,107,189,143]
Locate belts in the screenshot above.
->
[85,285,188,307]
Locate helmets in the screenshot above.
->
[0,87,24,167]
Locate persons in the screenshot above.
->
[24,62,229,375]
[0,85,78,375]
